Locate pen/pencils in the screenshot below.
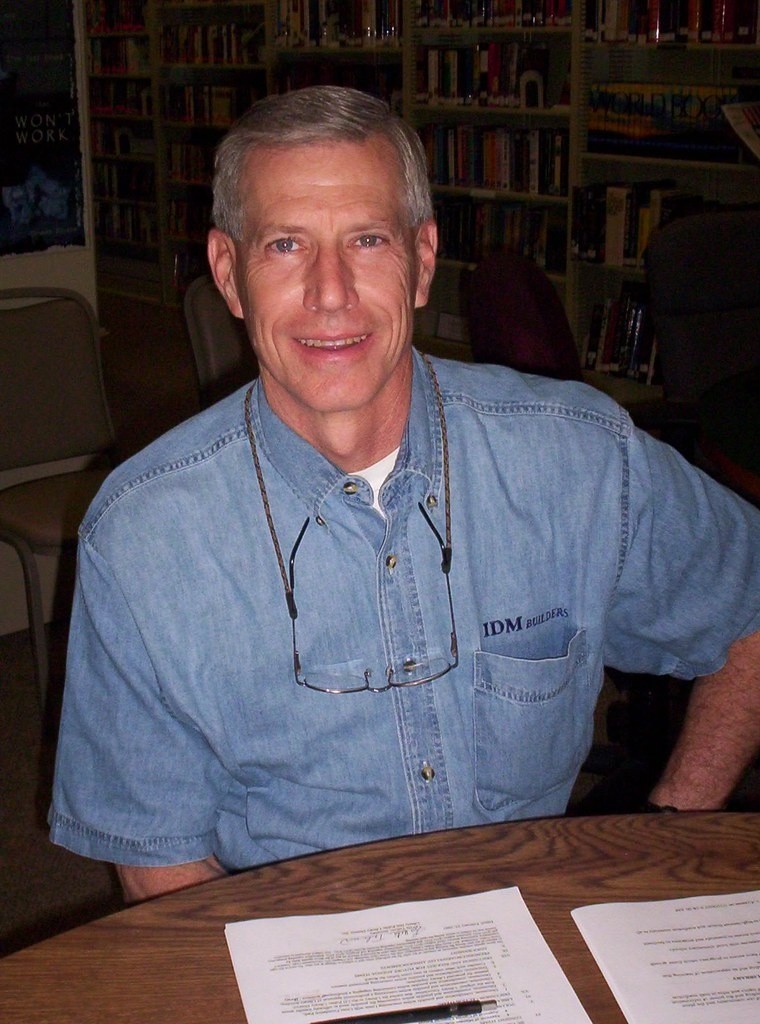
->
[311,999,497,1024]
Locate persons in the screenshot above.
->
[43,84,760,904]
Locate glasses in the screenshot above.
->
[290,503,461,695]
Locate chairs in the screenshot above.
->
[0,284,123,707]
[641,212,760,396]
[180,274,259,406]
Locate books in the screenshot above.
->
[81,0,760,391]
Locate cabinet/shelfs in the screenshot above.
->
[90,0,759,380]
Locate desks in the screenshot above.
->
[0,810,759,1024]
